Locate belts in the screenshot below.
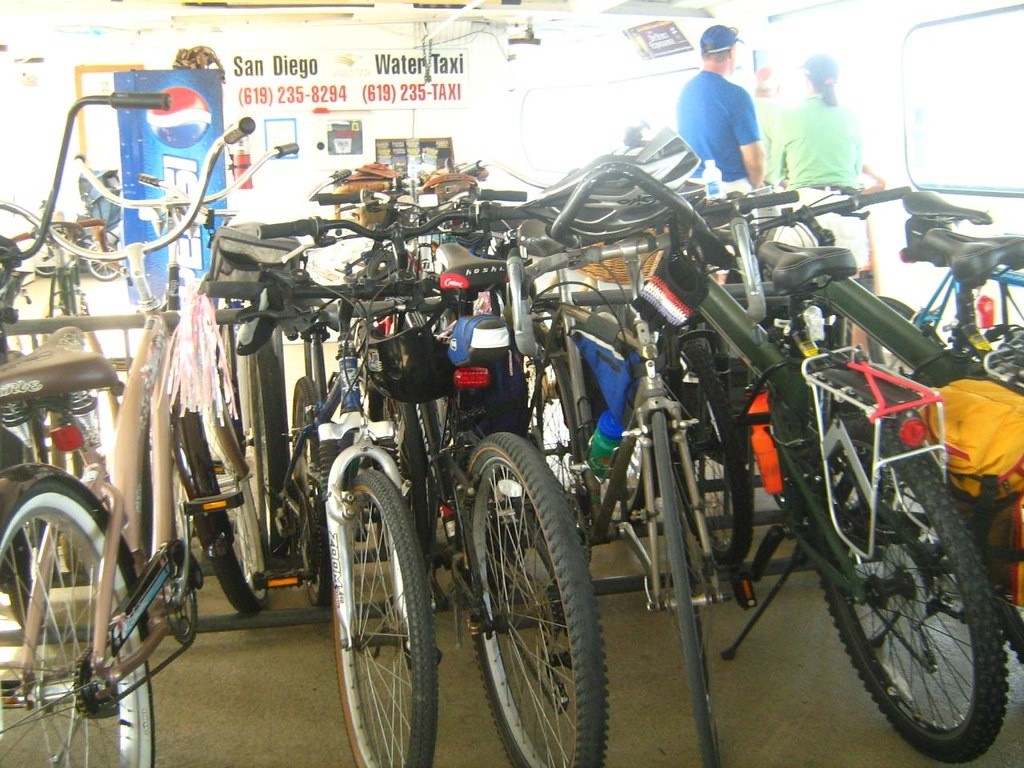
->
[814,185,856,198]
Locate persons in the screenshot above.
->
[676,23,767,286]
[771,55,877,360]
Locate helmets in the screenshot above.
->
[362,325,453,405]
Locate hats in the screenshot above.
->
[803,53,837,83]
[702,25,744,55]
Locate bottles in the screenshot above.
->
[702,159,726,202]
[744,383,784,493]
[585,410,625,479]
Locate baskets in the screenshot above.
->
[574,228,668,288]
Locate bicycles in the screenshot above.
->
[0,91,1023,768]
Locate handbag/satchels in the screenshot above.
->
[921,378,1024,607]
